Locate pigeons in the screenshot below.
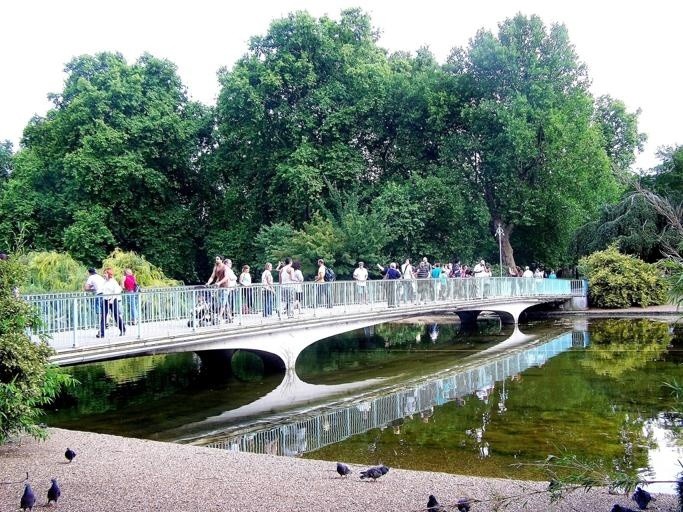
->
[456,497,471,512]
[425,493,441,511]
[358,465,389,484]
[64,446,77,464]
[18,482,37,512]
[628,485,658,508]
[44,477,61,509]
[335,462,353,478]
[609,502,634,512]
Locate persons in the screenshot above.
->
[118,267,136,325]
[0,253,41,348]
[384,340,392,354]
[84,267,110,329]
[509,265,557,296]
[428,323,440,344]
[416,334,421,345]
[95,268,127,338]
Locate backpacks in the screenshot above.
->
[324,267,336,282]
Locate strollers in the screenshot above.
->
[187,284,221,328]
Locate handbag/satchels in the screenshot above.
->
[379,274,389,287]
[135,284,141,292]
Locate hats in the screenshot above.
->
[526,266,530,270]
[481,260,485,265]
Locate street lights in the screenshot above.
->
[495,223,505,278]
